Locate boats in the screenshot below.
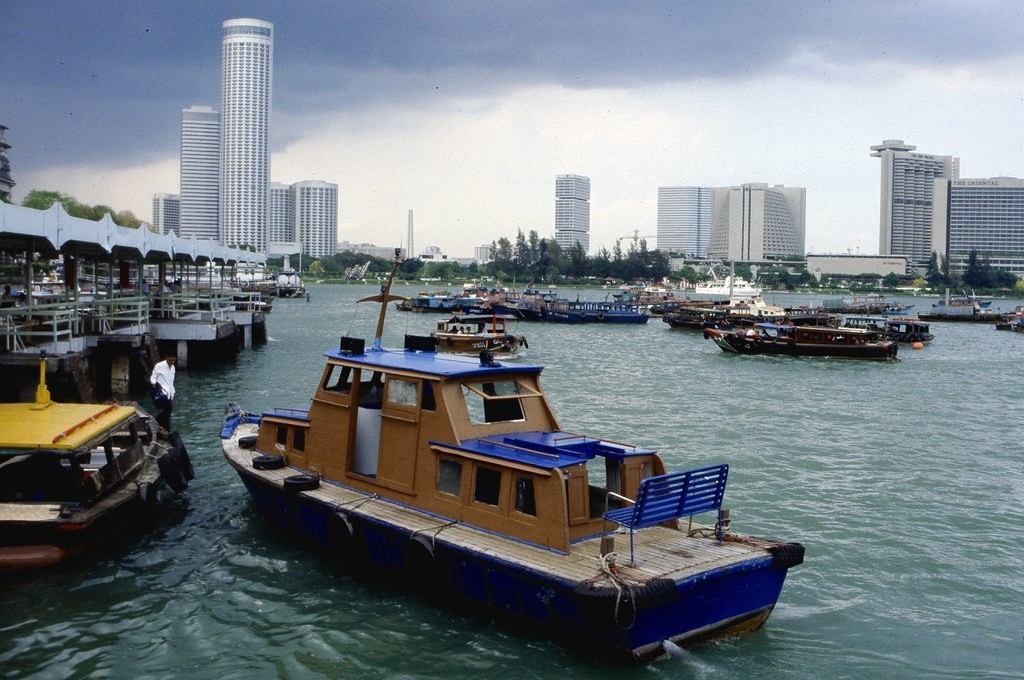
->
[405,315,529,356]
[221,246,806,672]
[396,258,1023,370]
[0,349,195,570]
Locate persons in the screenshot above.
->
[449,325,473,334]
[0,285,27,308]
[148,353,176,431]
[151,278,182,318]
[479,323,488,334]
[139,279,149,296]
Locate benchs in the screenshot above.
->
[599,463,731,566]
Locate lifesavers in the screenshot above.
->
[239,436,256,449]
[284,475,321,491]
[253,455,285,469]
